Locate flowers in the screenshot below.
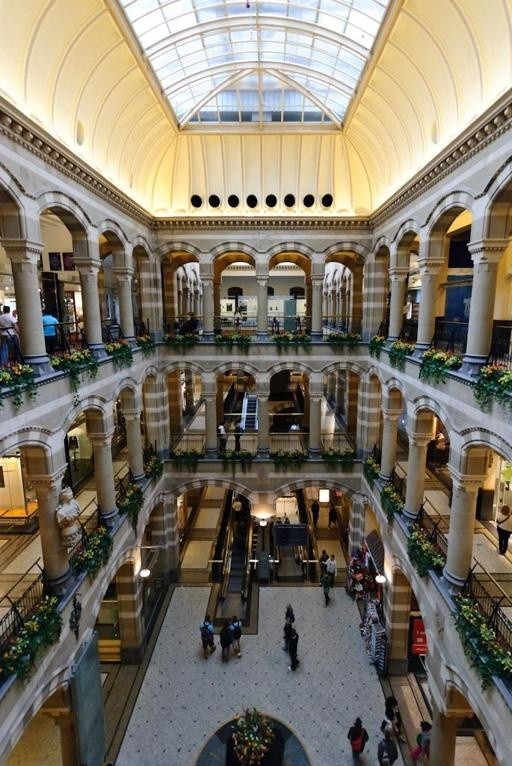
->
[215,333,251,355]
[499,370,512,415]
[419,349,435,378]
[15,362,40,402]
[52,357,82,408]
[473,364,498,415]
[169,446,356,474]
[368,334,384,361]
[0,367,24,412]
[163,334,201,355]
[326,333,363,356]
[0,457,162,686]
[137,335,156,360]
[231,705,272,763]
[388,341,415,374]
[363,458,511,692]
[272,333,313,355]
[105,342,134,372]
[69,351,84,382]
[437,357,464,387]
[81,349,99,380]
[430,352,448,385]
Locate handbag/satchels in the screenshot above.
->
[201,622,210,641]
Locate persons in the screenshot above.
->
[219,423,229,452]
[234,419,242,450]
[436,430,445,459]
[281,603,300,670]
[178,312,199,337]
[319,550,337,608]
[40,305,59,353]
[234,306,243,320]
[347,716,369,761]
[378,697,402,766]
[0,306,22,363]
[281,512,290,524]
[57,487,81,548]
[311,499,320,526]
[231,498,242,511]
[413,721,432,766]
[220,613,244,662]
[199,615,217,660]
[496,505,512,555]
[328,504,338,530]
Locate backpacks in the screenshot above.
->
[381,750,390,766]
[232,621,241,640]
[352,736,364,752]
